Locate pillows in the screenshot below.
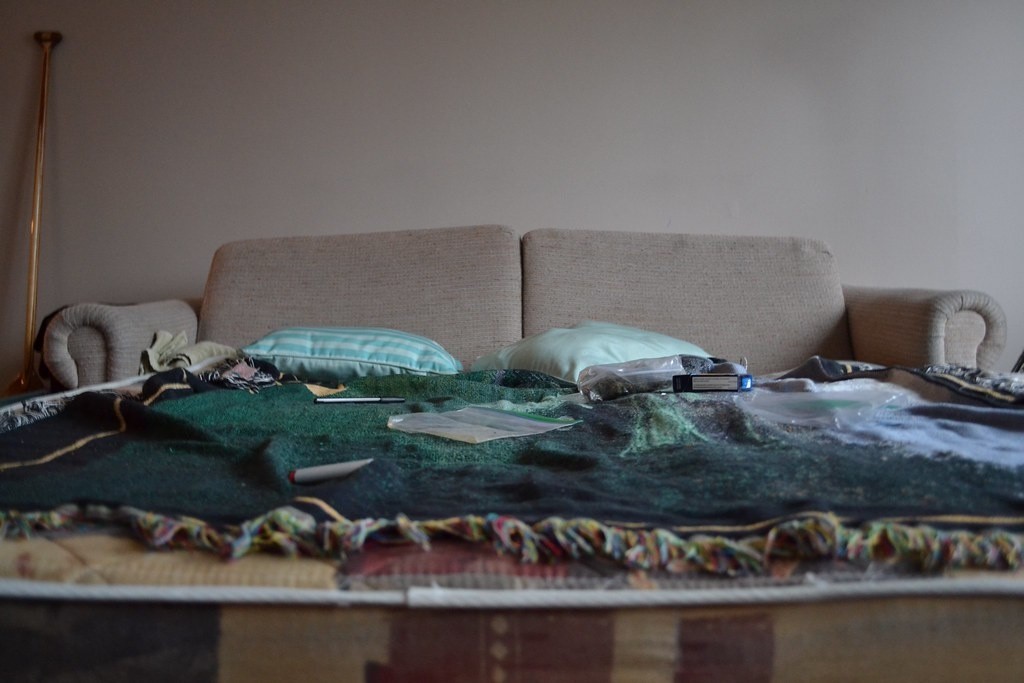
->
[242,327,462,380]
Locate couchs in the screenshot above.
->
[39,220,1007,386]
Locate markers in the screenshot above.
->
[289,457,372,483]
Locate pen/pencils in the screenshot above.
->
[314,397,406,404]
